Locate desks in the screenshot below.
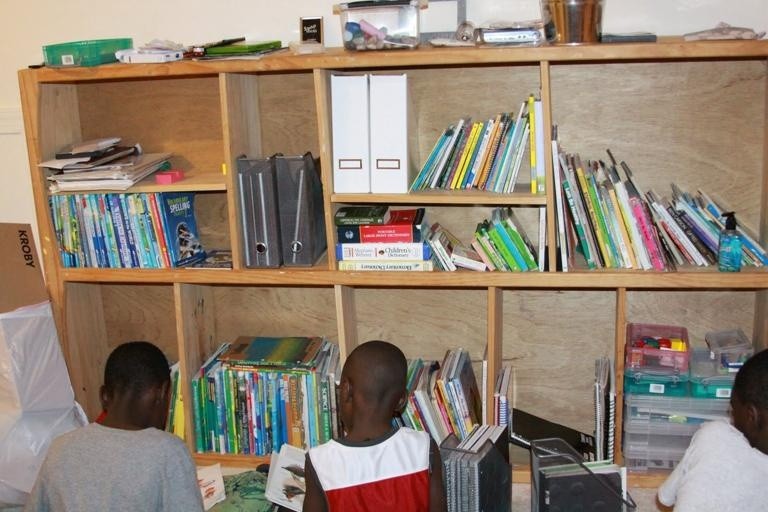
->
[191,464,665,512]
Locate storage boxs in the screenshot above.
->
[1,222,75,444]
[328,0,428,51]
[624,321,758,477]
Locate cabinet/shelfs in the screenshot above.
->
[16,34,768,488]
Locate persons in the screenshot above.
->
[25,341,206,511]
[656,350,767,512]
[282,341,448,511]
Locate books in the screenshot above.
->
[409,93,547,197]
[38,135,209,270]
[401,349,629,511]
[549,123,766,270]
[333,202,546,271]
[173,335,343,458]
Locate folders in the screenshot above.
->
[369,73,412,193]
[330,74,370,194]
